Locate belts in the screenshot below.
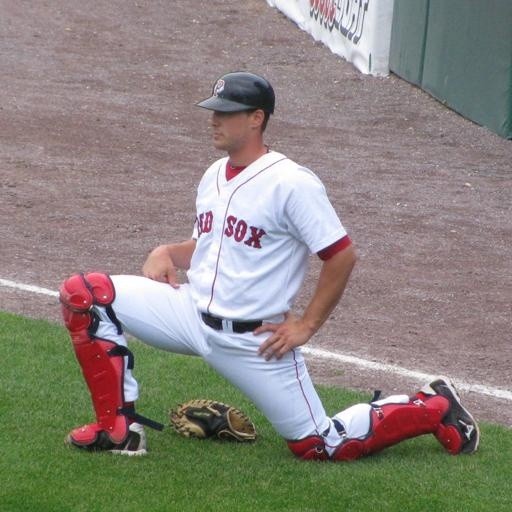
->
[201,312,262,333]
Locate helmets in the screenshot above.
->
[196,71,275,114]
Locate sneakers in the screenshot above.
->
[64,421,148,457]
[421,374,480,456]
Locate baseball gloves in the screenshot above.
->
[168,400,256,442]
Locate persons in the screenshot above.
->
[59,71,480,462]
